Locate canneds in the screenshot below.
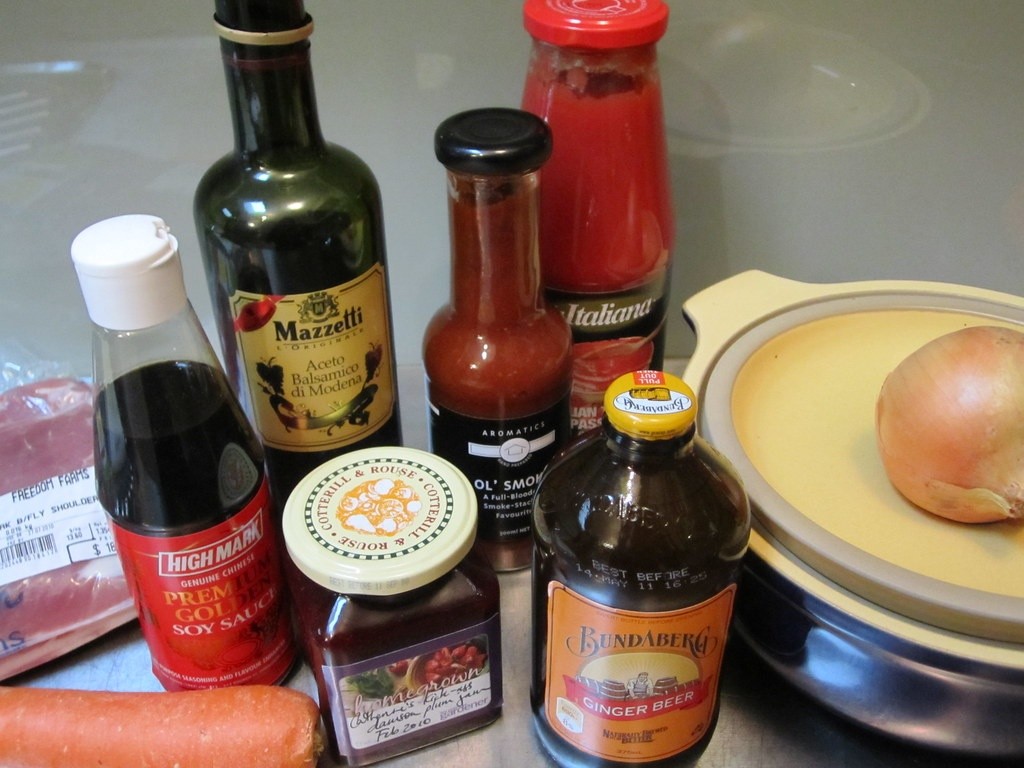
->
[283,443,503,766]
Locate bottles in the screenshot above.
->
[420,109,571,574]
[521,1,671,445]
[69,214,300,691]
[193,0,404,524]
[530,367,752,768]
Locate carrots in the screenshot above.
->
[0,682,324,767]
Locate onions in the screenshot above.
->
[875,325,1024,524]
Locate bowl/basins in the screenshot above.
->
[684,266,1024,762]
